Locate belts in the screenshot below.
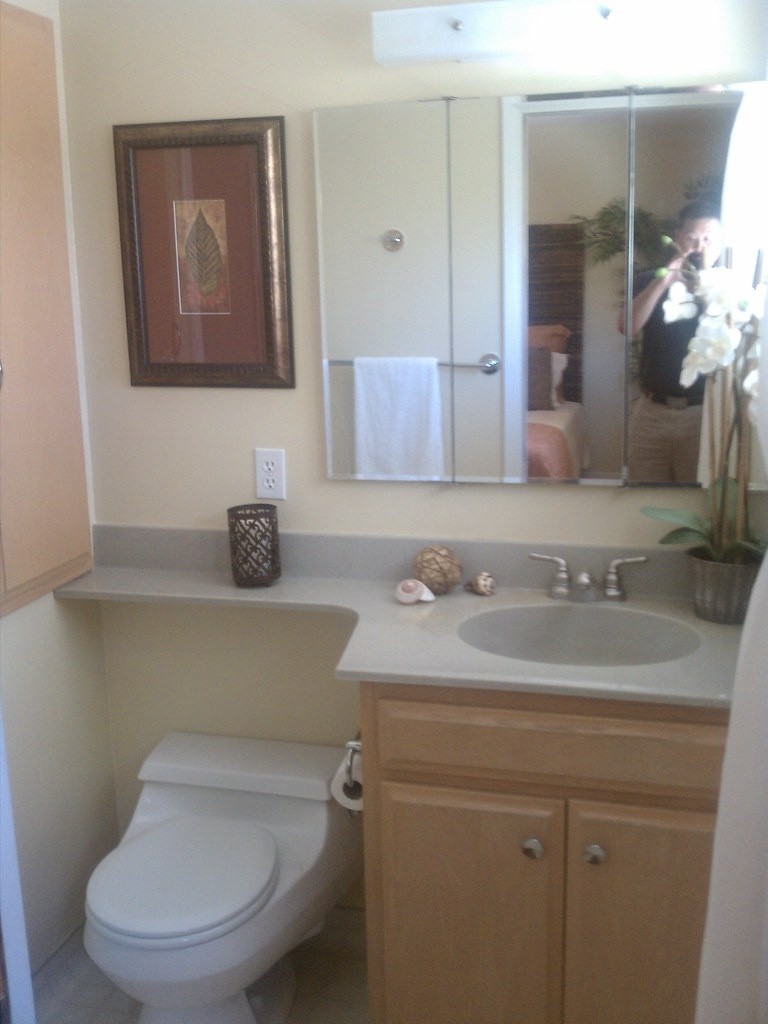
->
[640,386,703,409]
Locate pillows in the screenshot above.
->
[551,352,570,408]
[527,348,555,412]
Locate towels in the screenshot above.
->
[692,543,768,1024]
[694,361,740,492]
[352,356,444,479]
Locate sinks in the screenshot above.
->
[456,604,704,669]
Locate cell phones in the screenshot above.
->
[688,252,704,273]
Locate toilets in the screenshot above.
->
[81,730,364,1024]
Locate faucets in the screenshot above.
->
[570,571,605,602]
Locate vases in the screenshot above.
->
[667,517,765,626]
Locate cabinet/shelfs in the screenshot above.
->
[359,681,731,1024]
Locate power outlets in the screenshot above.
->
[255,447,287,500]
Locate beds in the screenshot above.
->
[526,323,589,482]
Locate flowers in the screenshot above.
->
[662,259,761,479]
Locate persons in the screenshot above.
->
[615,198,725,482]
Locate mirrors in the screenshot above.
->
[312,82,768,490]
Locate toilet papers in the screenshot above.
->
[330,751,364,811]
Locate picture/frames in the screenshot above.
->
[111,115,296,386]
